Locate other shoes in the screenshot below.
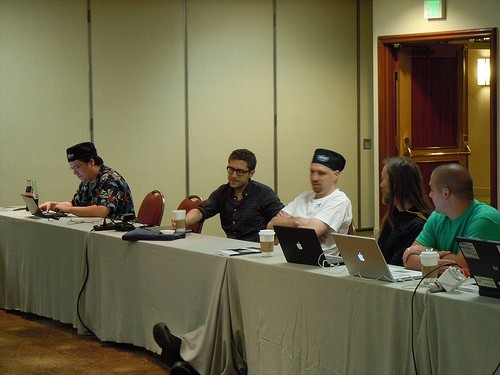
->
[169,362,200,375]
[153,322,182,366]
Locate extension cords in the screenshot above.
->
[93,222,129,230]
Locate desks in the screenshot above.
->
[0,207,500,375]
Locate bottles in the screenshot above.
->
[25,179,32,211]
[31,181,39,206]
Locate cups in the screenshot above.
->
[172,209,186,233]
[258,230,276,257]
[419,251,440,287]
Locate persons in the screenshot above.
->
[152,148,285,375]
[267,148,353,256]
[403,164,500,276]
[372,157,434,267]
[39,142,136,223]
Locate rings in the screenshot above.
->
[407,248,410,251]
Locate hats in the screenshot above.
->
[67,142,97,162]
[312,148,346,172]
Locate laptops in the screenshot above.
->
[455,236,500,298]
[330,233,423,283]
[273,224,345,267]
[21,194,68,218]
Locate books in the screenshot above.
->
[213,247,262,256]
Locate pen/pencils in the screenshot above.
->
[13,208,26,211]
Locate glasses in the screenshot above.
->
[227,166,251,176]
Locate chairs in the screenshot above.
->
[137,190,166,227]
[177,195,202,234]
